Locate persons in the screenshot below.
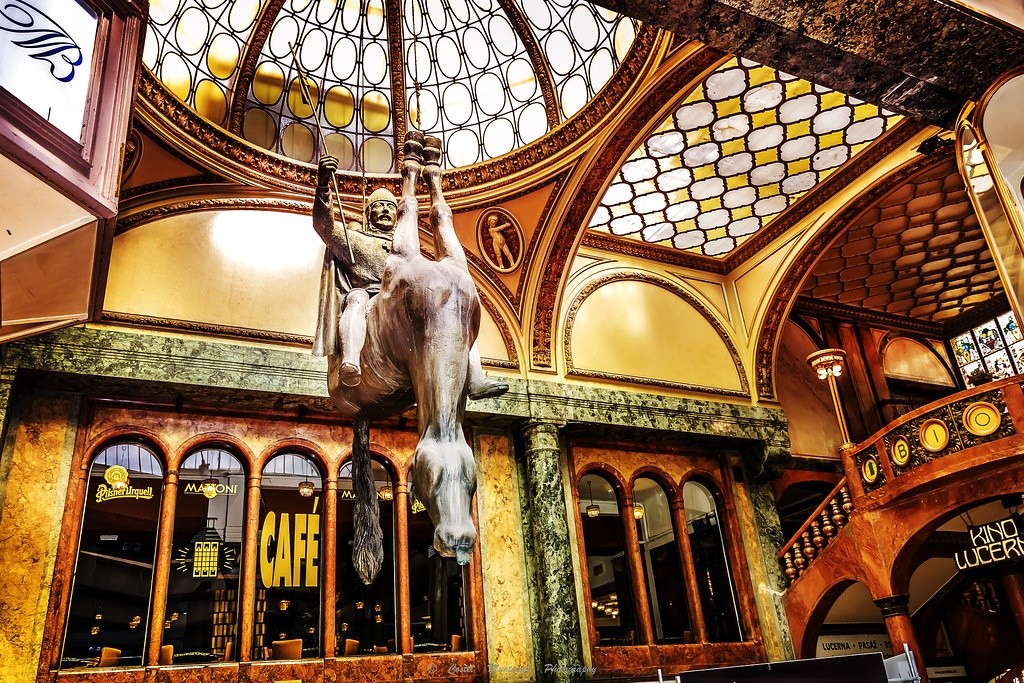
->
[313,154,509,400]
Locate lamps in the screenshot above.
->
[380,472,393,501]
[203,469,219,498]
[299,476,315,497]
[586,481,600,517]
[633,488,644,519]
[806,348,846,379]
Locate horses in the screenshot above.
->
[327,132,482,586]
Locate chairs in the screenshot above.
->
[452,635,461,652]
[160,644,174,665]
[272,638,302,659]
[95,647,122,666]
[345,639,359,655]
[373,646,387,654]
[224,642,232,661]
